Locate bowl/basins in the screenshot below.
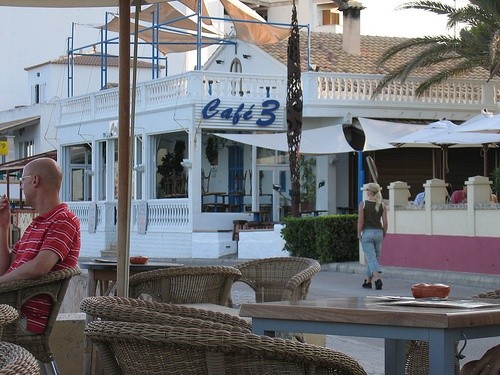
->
[411,286,450,301]
[130,256,149,264]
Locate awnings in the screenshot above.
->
[208,110,500,154]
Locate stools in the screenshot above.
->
[232,220,278,241]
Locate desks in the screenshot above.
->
[239,296,500,375]
[81,261,185,375]
[202,191,242,212]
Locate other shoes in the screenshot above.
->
[363,279,372,288]
[375,279,382,290]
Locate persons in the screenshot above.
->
[357,183,388,289]
[0,158,81,337]
[450,184,467,203]
[414,192,425,205]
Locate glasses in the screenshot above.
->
[19,175,33,184]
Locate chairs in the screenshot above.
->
[398,289,500,375]
[0,263,82,375]
[80,256,366,375]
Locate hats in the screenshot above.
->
[361,183,380,192]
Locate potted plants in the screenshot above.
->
[298,154,316,211]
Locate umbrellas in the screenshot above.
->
[389,116,500,186]
[415,108,500,178]
[448,113,500,133]
